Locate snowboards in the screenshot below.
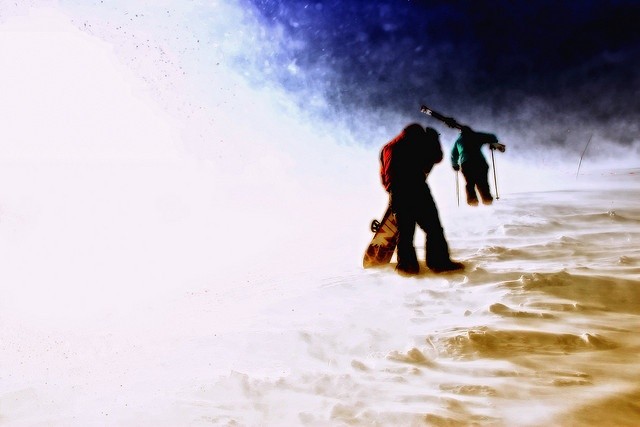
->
[363,200,399,268]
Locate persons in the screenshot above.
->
[451,125,498,205]
[379,124,465,273]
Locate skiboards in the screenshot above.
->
[420,105,506,153]
